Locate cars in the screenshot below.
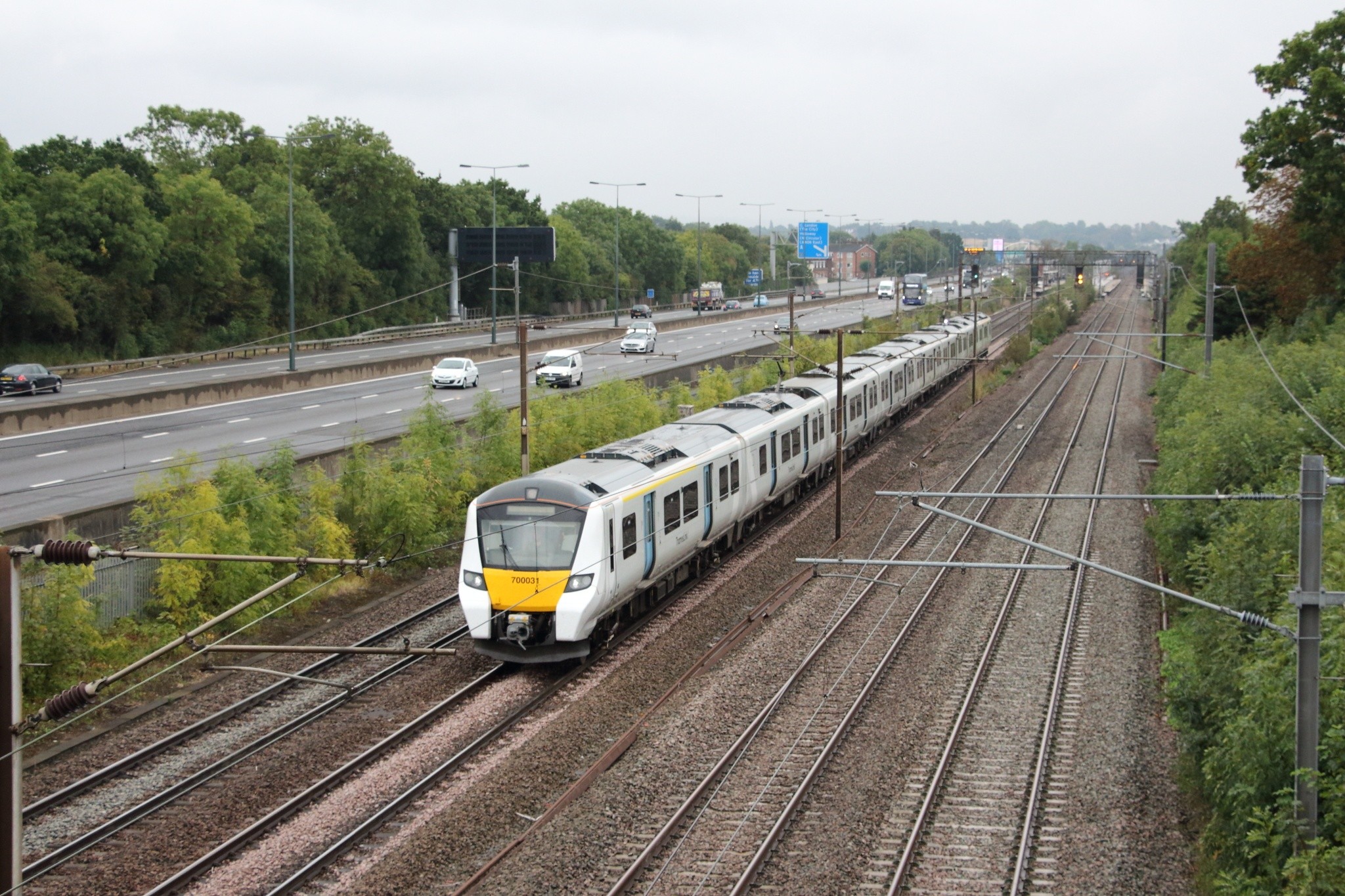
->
[875,270,991,305]
[631,304,652,318]
[810,290,825,299]
[774,317,799,334]
[723,295,767,311]
[0,363,65,396]
[620,322,657,353]
[430,357,479,389]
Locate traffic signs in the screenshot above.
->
[797,222,829,259]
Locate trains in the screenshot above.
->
[458,310,991,663]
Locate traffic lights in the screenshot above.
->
[972,265,979,286]
[1075,267,1083,285]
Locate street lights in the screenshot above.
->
[787,209,883,302]
[460,164,530,344]
[238,132,336,372]
[676,194,723,315]
[740,203,775,307]
[590,181,646,327]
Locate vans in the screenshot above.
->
[536,349,584,388]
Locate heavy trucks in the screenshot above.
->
[689,281,724,310]
